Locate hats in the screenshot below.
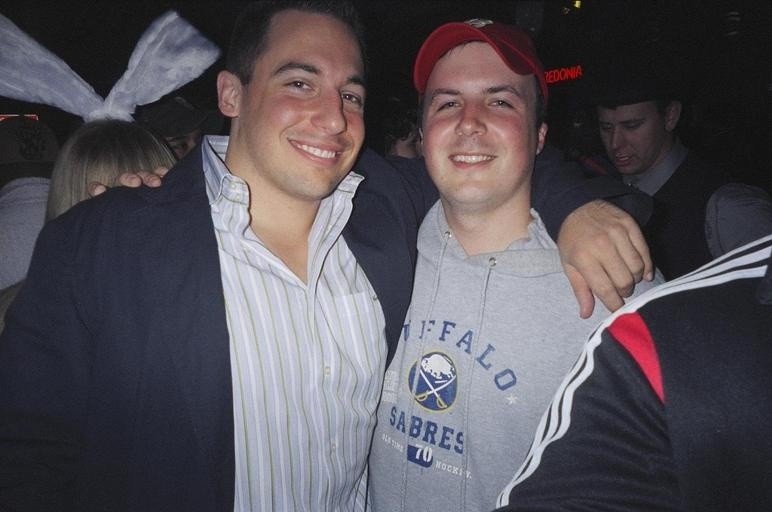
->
[580,68,673,108]
[410,15,553,107]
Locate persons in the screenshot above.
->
[0,110,62,324]
[595,62,772,280]
[87,16,665,508]
[134,88,229,165]
[1,0,655,510]
[365,85,428,164]
[486,237,772,512]
[9,116,181,324]
